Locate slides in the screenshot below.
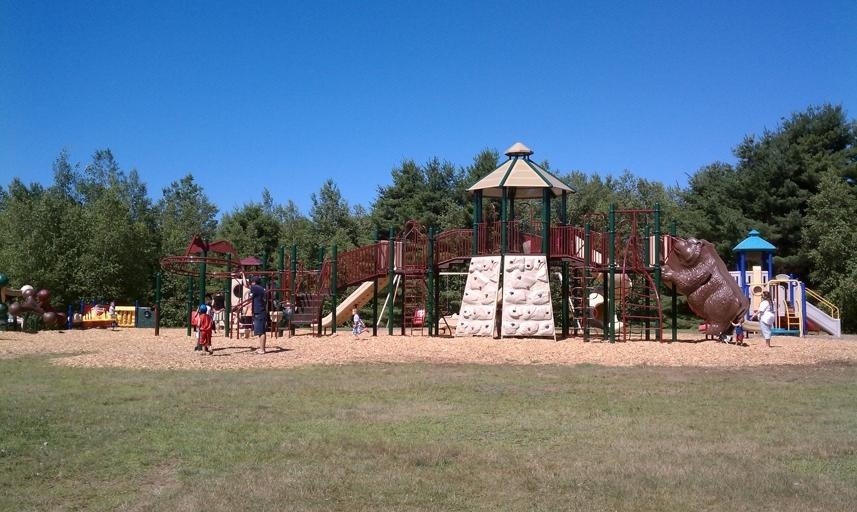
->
[575,237,632,332]
[310,276,391,328]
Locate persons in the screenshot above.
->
[240,269,267,354]
[729,317,745,345]
[750,291,775,347]
[351,308,370,340]
[194,294,217,356]
[7,300,24,329]
[94,301,119,314]
